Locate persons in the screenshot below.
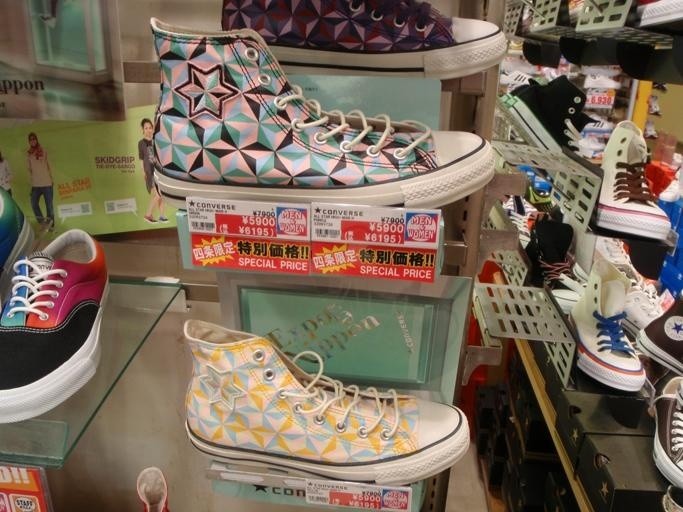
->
[0,151,13,198]
[26,132,54,232]
[137,117,169,224]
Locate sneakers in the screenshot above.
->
[184,320,470,487]
[222,0,507,78]
[651,376,682,490]
[499,75,682,392]
[149,17,494,213]
[0,186,108,424]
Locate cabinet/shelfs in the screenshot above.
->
[0,0,683,512]
